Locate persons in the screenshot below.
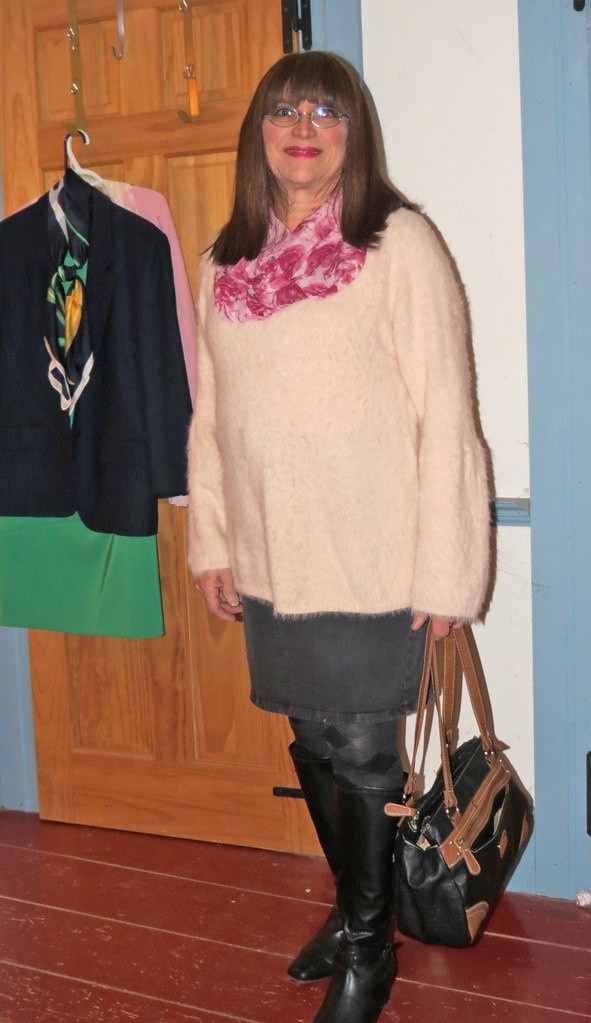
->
[186,53,490,1022]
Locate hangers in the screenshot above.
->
[31,126,140,194]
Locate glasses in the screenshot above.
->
[265,107,351,129]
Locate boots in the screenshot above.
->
[272,740,345,983]
[313,772,408,1023]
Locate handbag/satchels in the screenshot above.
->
[384,618,535,950]
